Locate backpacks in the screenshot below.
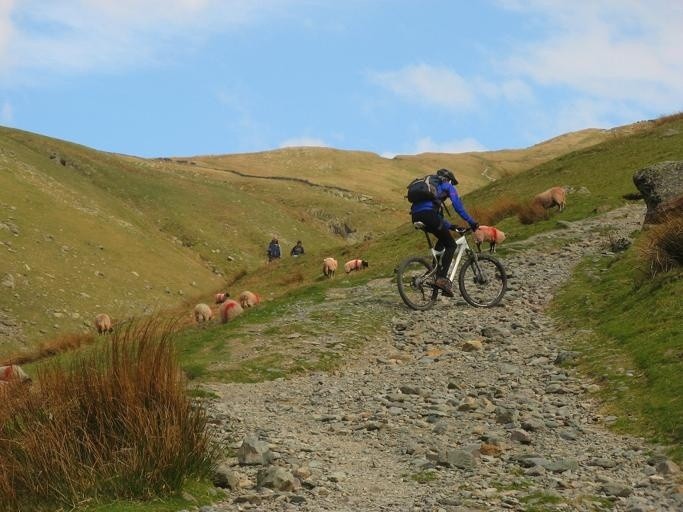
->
[407,176,444,206]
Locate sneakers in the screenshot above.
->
[435,278,454,296]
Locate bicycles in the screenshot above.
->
[395,220,508,311]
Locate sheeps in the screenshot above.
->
[214,293,230,304]
[193,302,212,322]
[342,259,369,275]
[322,257,338,278]
[0,363,33,388]
[95,313,113,336]
[239,289,258,310]
[218,298,243,322]
[471,224,508,253]
[531,187,566,212]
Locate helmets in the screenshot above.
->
[438,169,457,186]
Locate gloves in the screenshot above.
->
[449,224,458,230]
[471,223,479,231]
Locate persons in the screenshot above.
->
[408,168,479,297]
[289,240,304,257]
[266,236,281,262]
